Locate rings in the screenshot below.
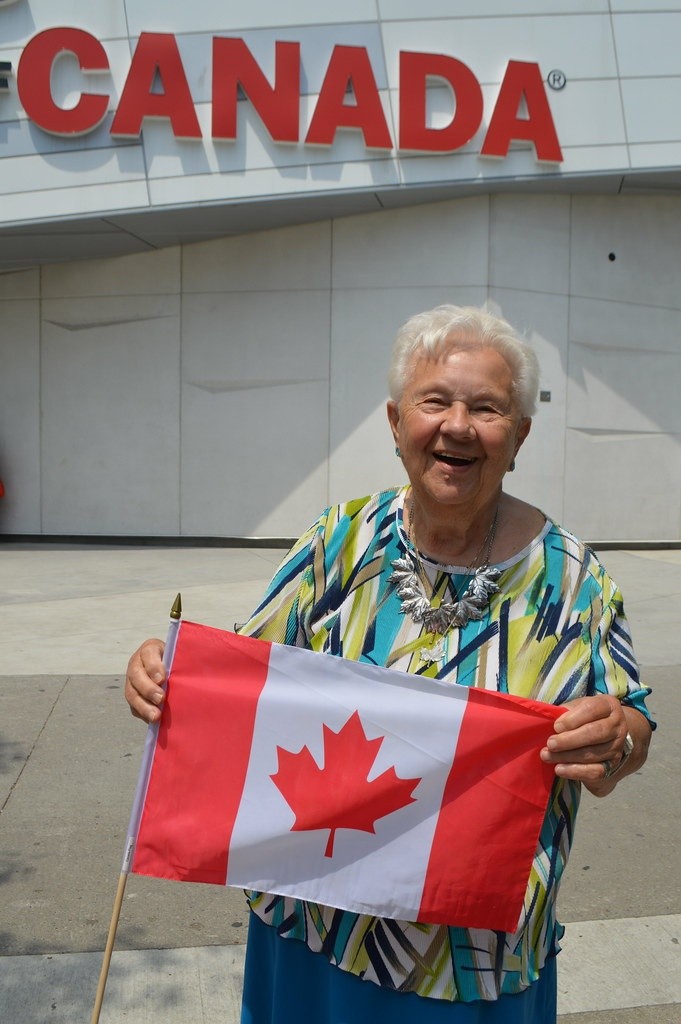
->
[603,760,610,779]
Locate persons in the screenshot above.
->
[127,305,656,1024]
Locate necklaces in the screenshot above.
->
[386,502,499,667]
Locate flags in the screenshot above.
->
[121,618,568,934]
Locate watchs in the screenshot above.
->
[611,734,634,776]
